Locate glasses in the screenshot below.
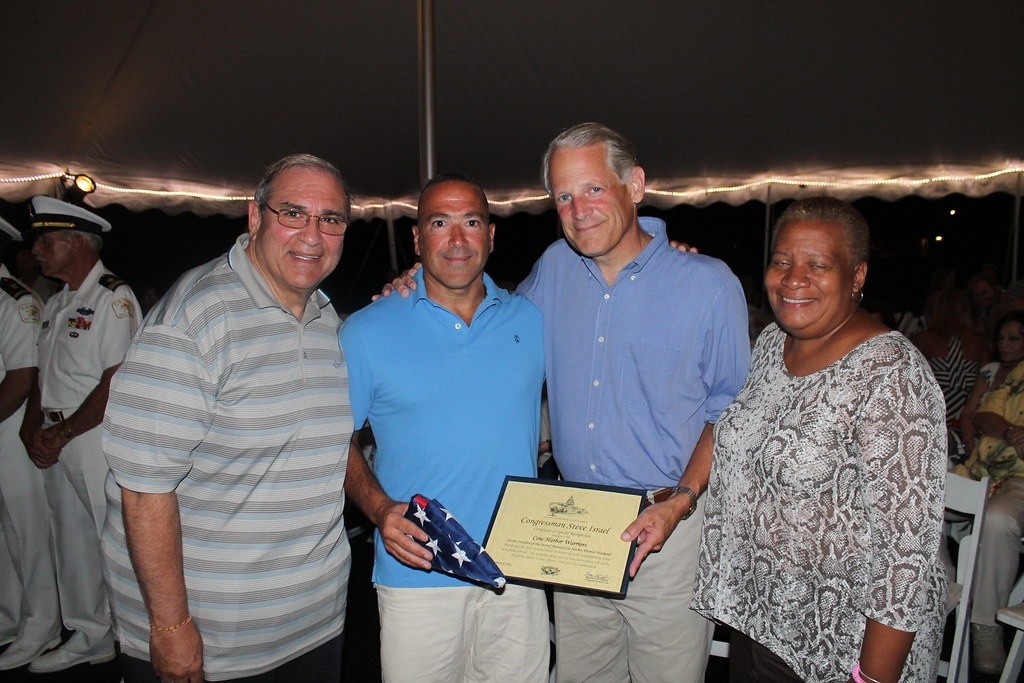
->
[261,198,348,236]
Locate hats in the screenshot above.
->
[0,218,22,242]
[20,195,112,237]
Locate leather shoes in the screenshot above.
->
[0,635,61,671]
[28,644,116,672]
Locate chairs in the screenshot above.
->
[708,471,1024,683]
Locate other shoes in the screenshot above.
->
[972,623,1005,669]
[0,634,17,646]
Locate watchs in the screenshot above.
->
[670,486,697,520]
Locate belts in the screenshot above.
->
[644,488,671,509]
[41,410,65,423]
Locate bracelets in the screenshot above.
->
[149,612,191,633]
[850,660,881,683]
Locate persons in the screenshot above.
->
[101,153,355,683]
[865,263,1024,683]
[337,168,552,683]
[0,194,144,674]
[368,120,752,683]
[663,194,956,683]
